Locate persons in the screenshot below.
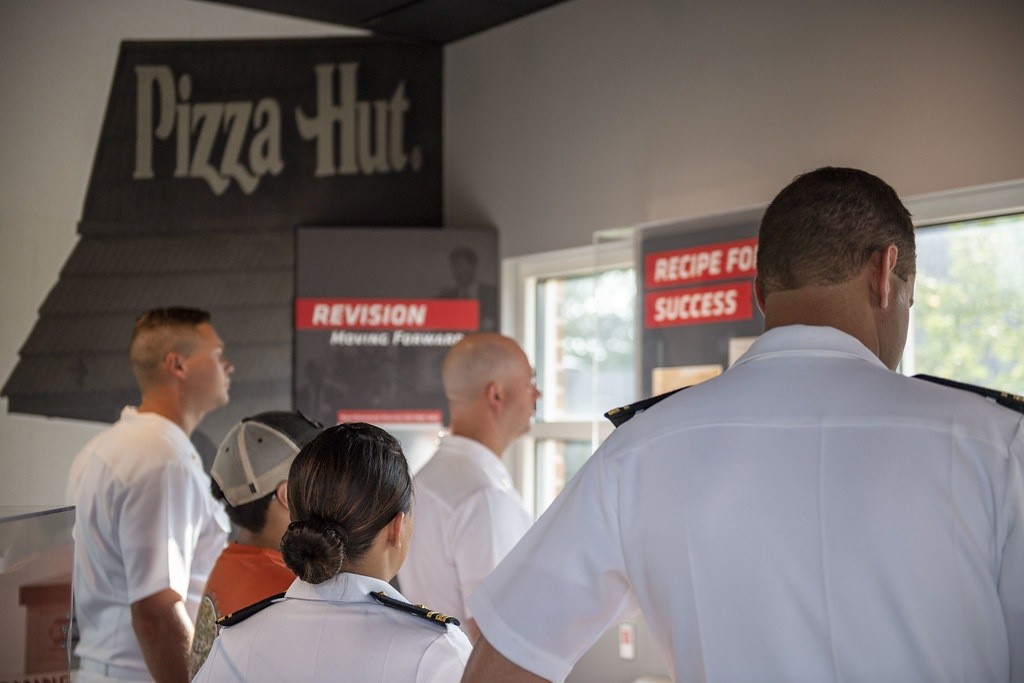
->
[461,167,1024,683]
[188,421,473,683]
[190,410,326,683]
[395,332,541,647]
[71,307,231,683]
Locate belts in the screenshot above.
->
[79,658,154,683]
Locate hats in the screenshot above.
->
[209,408,324,507]
